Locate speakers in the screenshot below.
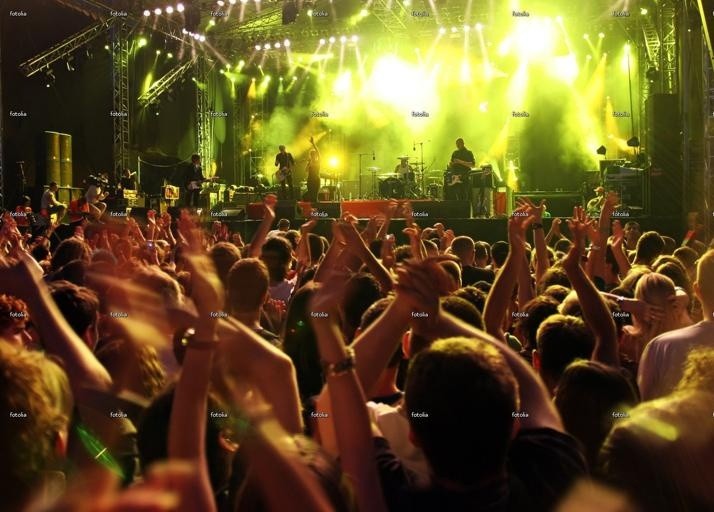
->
[317,201,341,219]
[274,199,304,219]
[437,199,473,218]
[219,209,245,220]
[35,130,84,213]
[642,92,685,217]
[409,200,438,217]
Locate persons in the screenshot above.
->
[303,135,321,201]
[274,145,297,199]
[0,174,714,511]
[182,154,206,206]
[394,160,413,173]
[447,137,476,200]
[117,169,136,197]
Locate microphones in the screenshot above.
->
[413,144,416,151]
[372,150,375,161]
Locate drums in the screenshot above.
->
[381,178,403,199]
[404,172,415,182]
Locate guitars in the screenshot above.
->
[275,158,306,182]
[367,168,381,171]
[397,157,410,160]
[187,177,220,191]
[409,163,425,165]
[447,168,491,186]
